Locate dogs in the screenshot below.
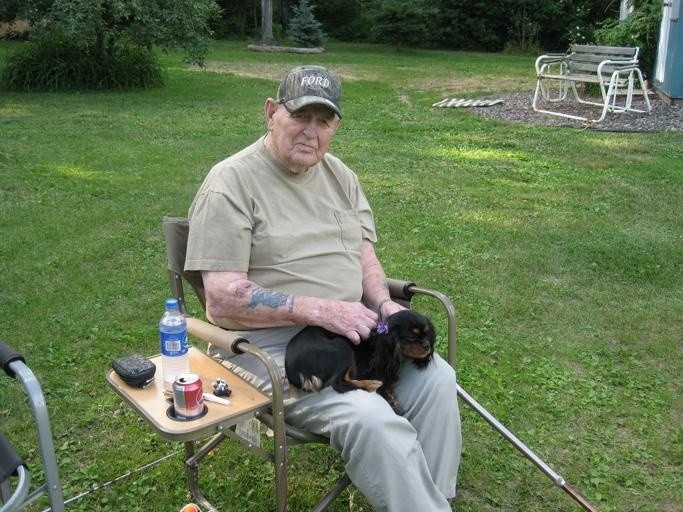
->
[284,309,436,415]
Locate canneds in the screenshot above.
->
[173,372,205,419]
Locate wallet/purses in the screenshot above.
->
[112,354,156,389]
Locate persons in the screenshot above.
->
[184,64,462,512]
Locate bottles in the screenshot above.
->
[160,299,190,394]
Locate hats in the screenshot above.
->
[276,64,343,120]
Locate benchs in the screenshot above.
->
[532,46,652,124]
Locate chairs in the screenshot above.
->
[1,343,69,511]
[162,218,458,511]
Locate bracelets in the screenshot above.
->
[378,298,392,310]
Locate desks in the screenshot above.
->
[106,342,272,445]
